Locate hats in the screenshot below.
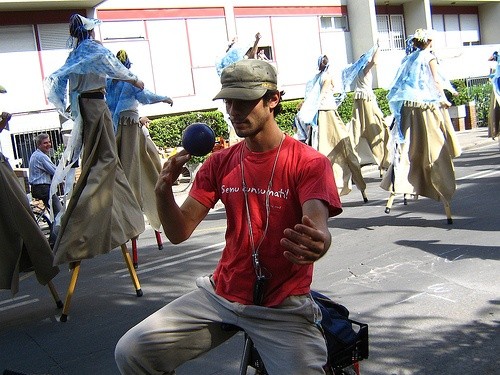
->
[213,59,277,100]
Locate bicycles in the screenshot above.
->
[159,150,193,194]
[31,179,76,241]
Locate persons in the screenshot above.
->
[343,37,395,187]
[0,111,62,293]
[45,14,145,264]
[306,53,367,197]
[292,98,312,143]
[28,133,66,250]
[114,58,343,375]
[401,35,459,97]
[214,35,247,147]
[257,50,269,60]
[385,28,462,205]
[106,49,174,231]
[244,32,262,61]
[488,48,500,138]
